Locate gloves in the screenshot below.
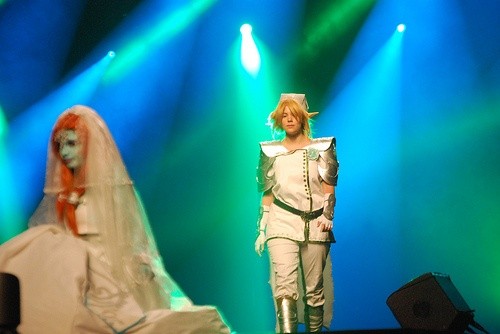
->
[255,205,269,256]
[317,193,335,232]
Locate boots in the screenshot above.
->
[305,304,324,334]
[276,298,297,334]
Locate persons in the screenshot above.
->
[255,92,341,334]
[0,105,230,333]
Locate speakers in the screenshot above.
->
[386,272,474,334]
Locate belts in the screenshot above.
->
[273,198,324,219]
